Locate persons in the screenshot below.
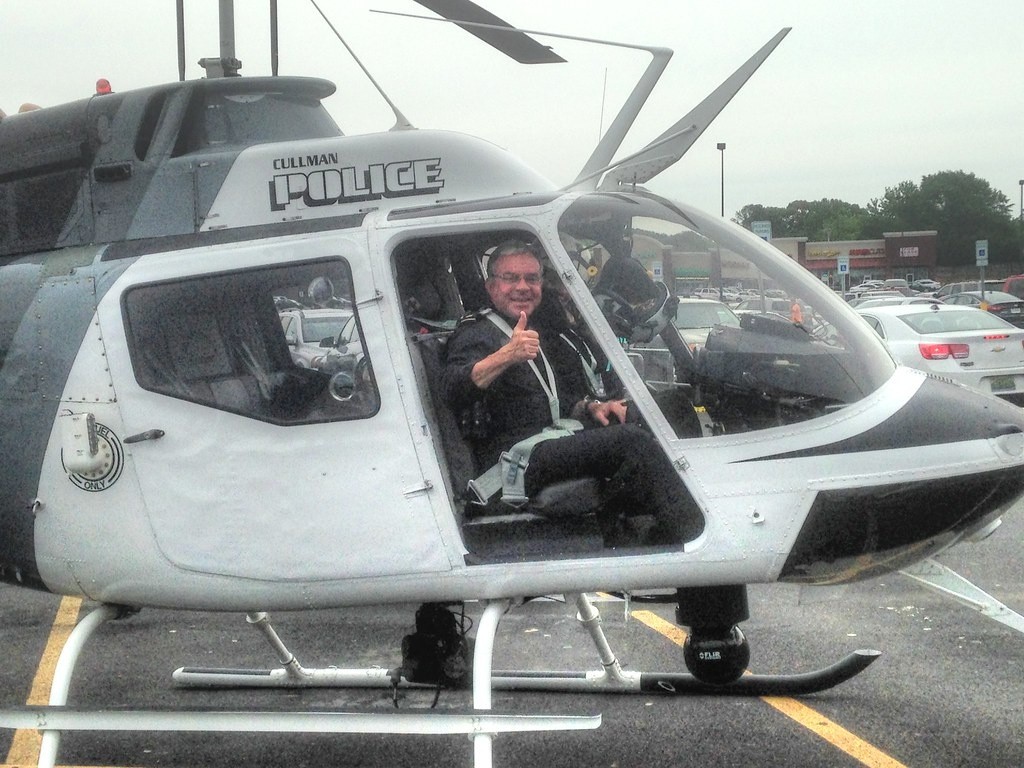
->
[790,295,804,324]
[439,231,706,545]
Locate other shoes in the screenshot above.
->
[601,503,700,545]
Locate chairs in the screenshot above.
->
[957,318,981,330]
[922,316,945,332]
[397,258,555,527]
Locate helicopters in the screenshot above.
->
[0,1,1024,740]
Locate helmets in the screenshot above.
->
[589,255,675,342]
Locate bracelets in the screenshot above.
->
[584,399,600,414]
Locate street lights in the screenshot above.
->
[714,141,726,303]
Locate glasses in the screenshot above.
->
[488,271,544,285]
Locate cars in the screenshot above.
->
[681,276,1023,399]
